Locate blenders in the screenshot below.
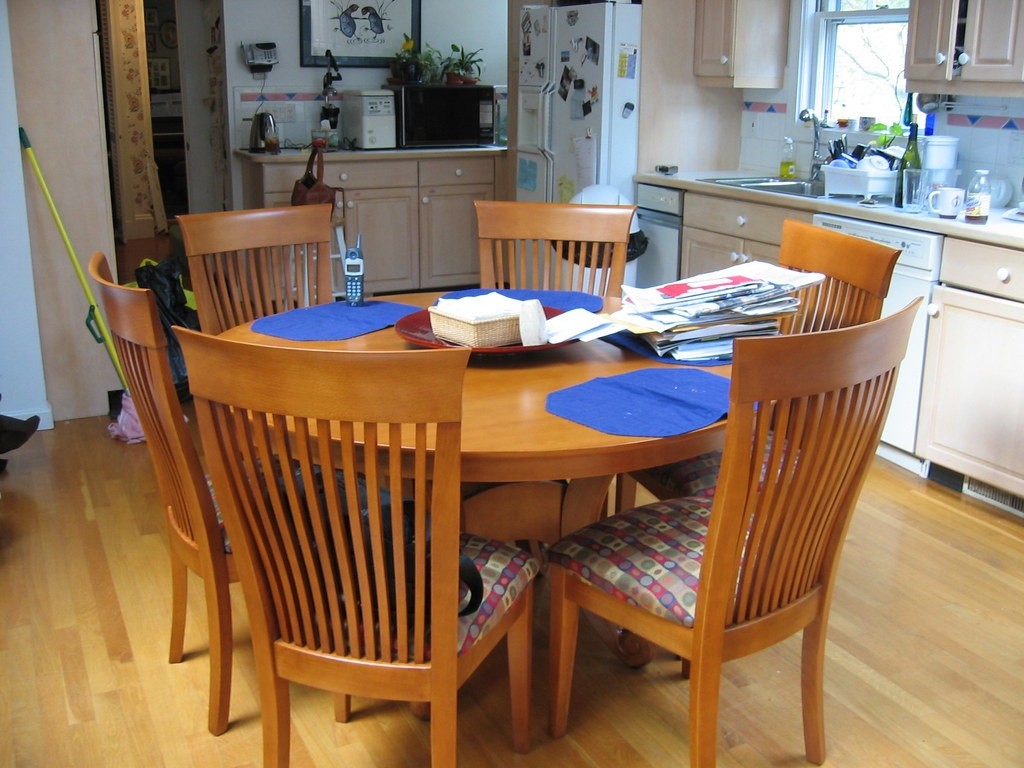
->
[320,49,350,152]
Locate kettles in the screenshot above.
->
[249,113,277,153]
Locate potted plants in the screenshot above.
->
[444,46,486,84]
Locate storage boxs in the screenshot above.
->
[820,161,897,203]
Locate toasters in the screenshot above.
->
[344,88,396,149]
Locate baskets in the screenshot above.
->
[428,306,521,348]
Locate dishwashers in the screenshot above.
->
[804,214,945,455]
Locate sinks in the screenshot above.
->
[695,176,804,186]
[741,183,825,198]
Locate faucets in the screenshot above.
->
[798,108,848,180]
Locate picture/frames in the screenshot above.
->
[300,0,423,69]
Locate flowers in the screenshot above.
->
[396,33,424,61]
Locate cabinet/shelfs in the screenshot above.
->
[678,193,816,279]
[242,153,510,298]
[915,221,1024,500]
[692,0,790,89]
[903,0,1024,98]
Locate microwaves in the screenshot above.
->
[381,84,497,148]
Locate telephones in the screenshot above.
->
[240,42,281,67]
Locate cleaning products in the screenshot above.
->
[779,136,798,181]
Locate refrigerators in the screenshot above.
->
[517,2,643,298]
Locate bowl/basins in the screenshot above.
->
[856,156,891,171]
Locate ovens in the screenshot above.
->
[635,208,683,289]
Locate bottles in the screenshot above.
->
[779,136,797,181]
[965,170,991,225]
[894,123,922,208]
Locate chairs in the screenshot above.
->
[167,318,541,768]
[173,200,341,331]
[472,199,640,298]
[79,242,430,740]
[542,288,929,768]
[614,219,904,514]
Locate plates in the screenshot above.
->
[395,306,580,359]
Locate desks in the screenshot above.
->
[197,287,836,719]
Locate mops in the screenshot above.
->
[18,126,189,446]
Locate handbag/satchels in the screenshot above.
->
[291,147,336,221]
[306,501,484,630]
[135,259,201,404]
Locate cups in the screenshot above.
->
[928,187,965,219]
[902,169,929,214]
[859,117,875,132]
[265,127,280,155]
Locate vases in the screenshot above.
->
[391,59,423,82]
